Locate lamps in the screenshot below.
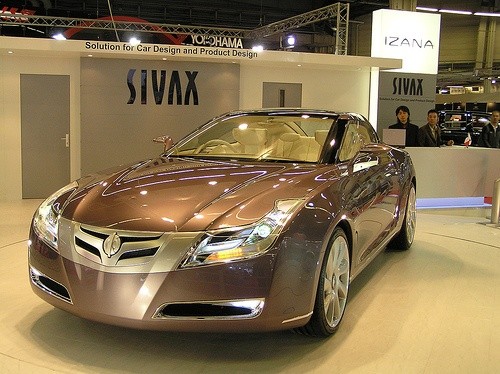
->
[282,32,296,48]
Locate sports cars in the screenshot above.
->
[28,107,417,336]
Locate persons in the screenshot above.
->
[388,105,419,147]
[478,110,500,148]
[418,109,454,147]
